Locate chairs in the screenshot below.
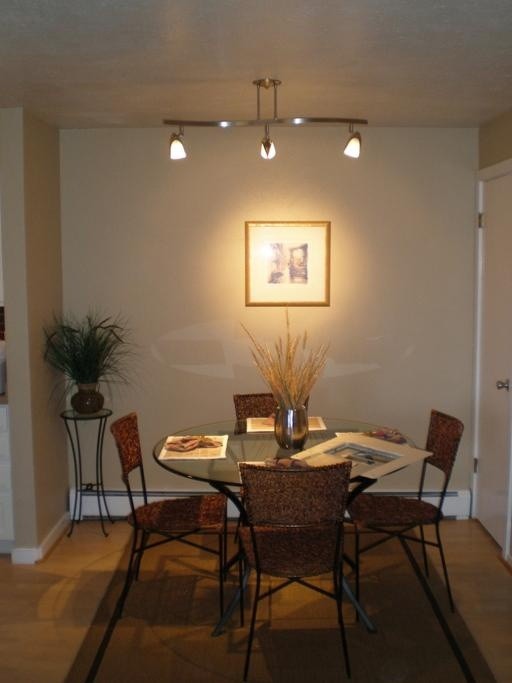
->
[238,461,355,683]
[108,412,229,625]
[337,409,465,622]
[233,393,310,545]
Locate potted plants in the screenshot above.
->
[41,303,146,415]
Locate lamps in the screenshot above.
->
[163,119,370,161]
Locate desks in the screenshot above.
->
[153,416,416,637]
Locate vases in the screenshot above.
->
[274,408,308,450]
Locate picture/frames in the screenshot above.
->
[244,221,331,308]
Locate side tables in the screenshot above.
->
[60,408,115,538]
